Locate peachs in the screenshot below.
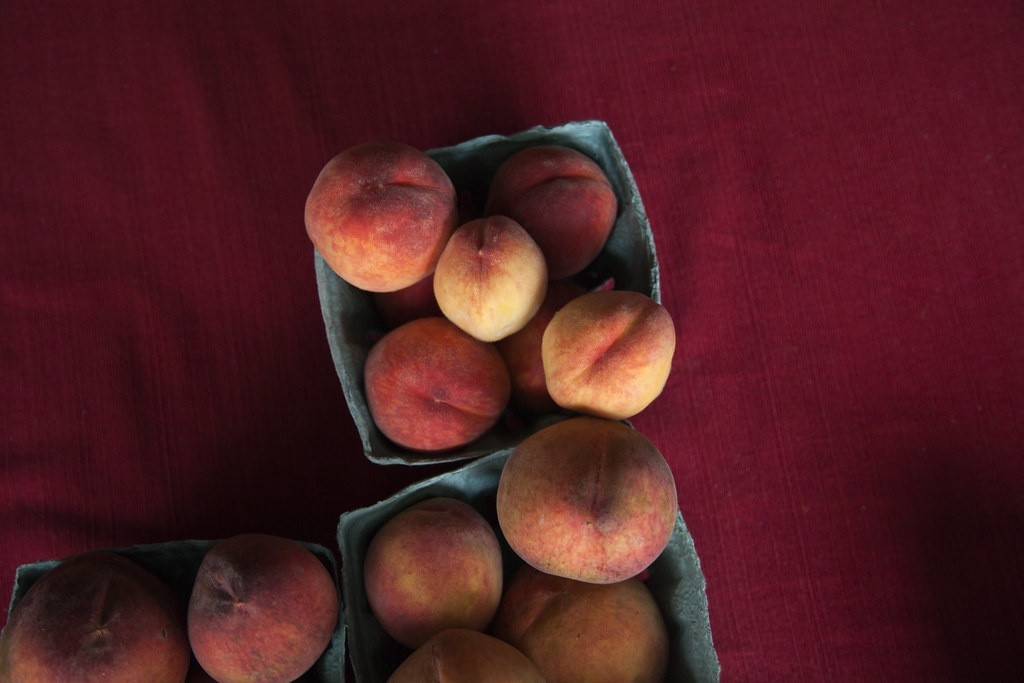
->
[3,138,678,682]
